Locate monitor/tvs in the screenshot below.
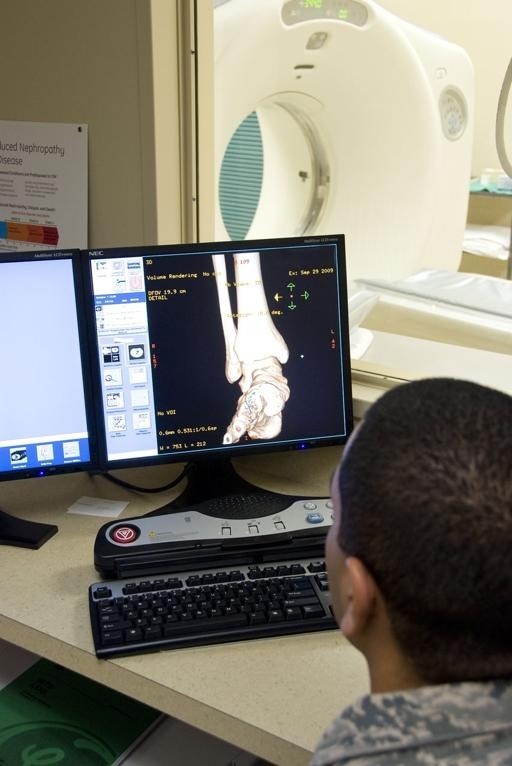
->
[0,248,99,549]
[80,233,354,578]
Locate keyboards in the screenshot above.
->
[88,557,339,659]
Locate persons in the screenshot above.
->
[301,377,512,766]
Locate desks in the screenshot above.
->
[0,445,371,766]
